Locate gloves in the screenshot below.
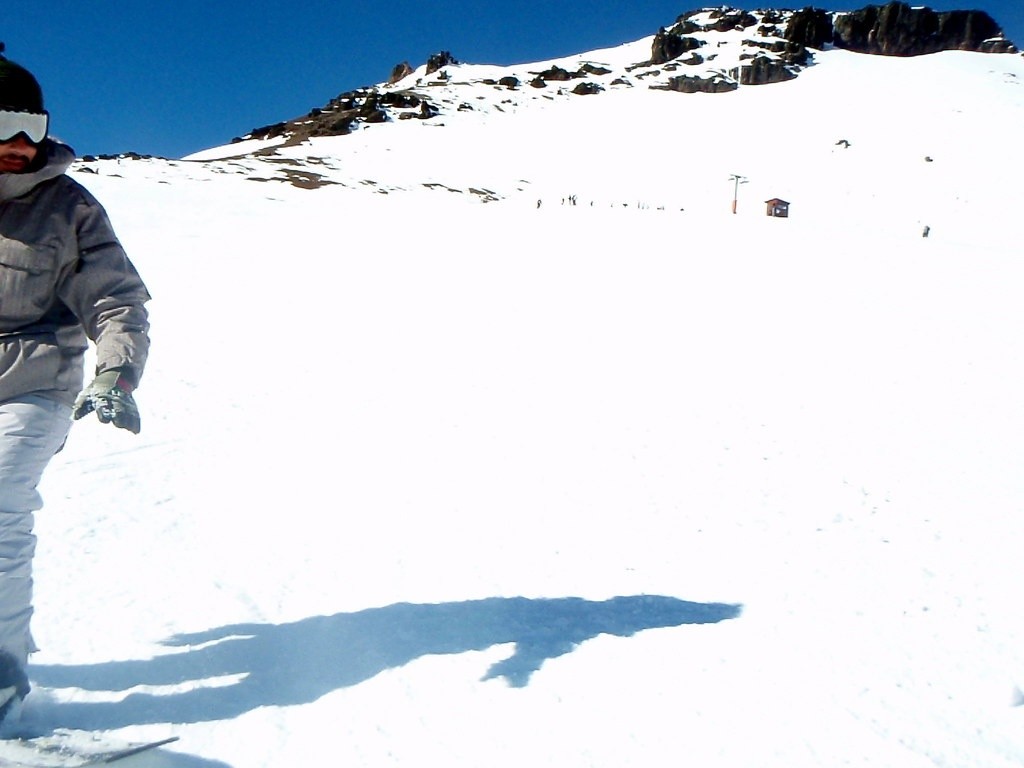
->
[73,368,140,434]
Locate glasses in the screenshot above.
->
[0,110,47,144]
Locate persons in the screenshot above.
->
[1,55,151,723]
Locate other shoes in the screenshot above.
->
[0,652,31,720]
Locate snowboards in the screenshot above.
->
[1,719,181,767]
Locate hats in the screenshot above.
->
[0,41,49,148]
